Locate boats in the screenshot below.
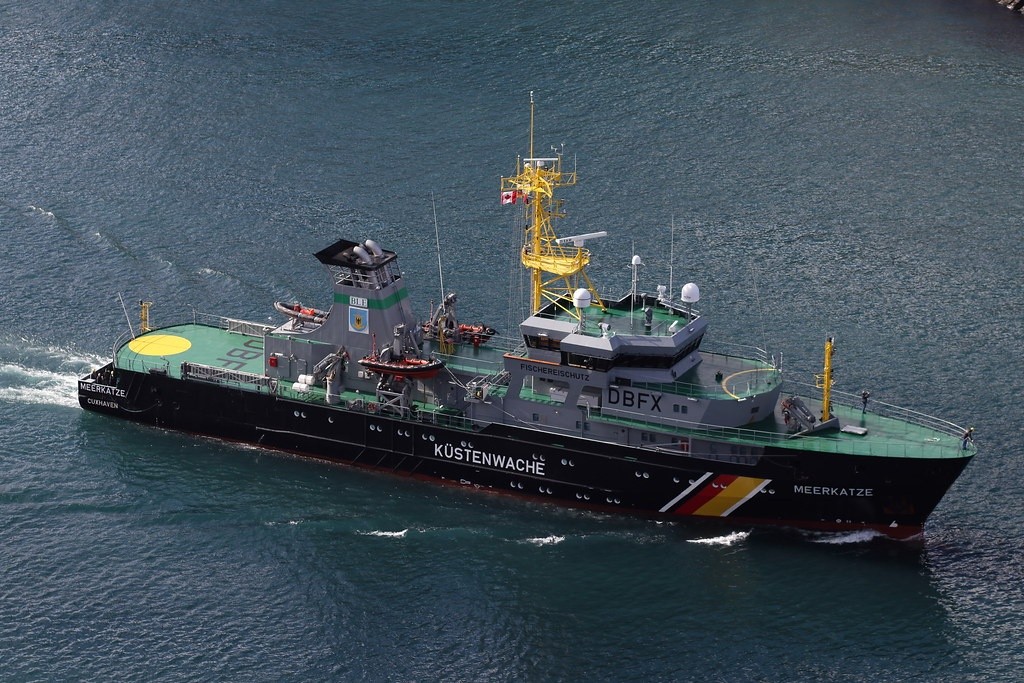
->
[77,87,980,538]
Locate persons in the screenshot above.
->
[861,390,870,414]
[92,368,119,388]
[962,427,974,450]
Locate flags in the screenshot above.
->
[501,190,516,205]
